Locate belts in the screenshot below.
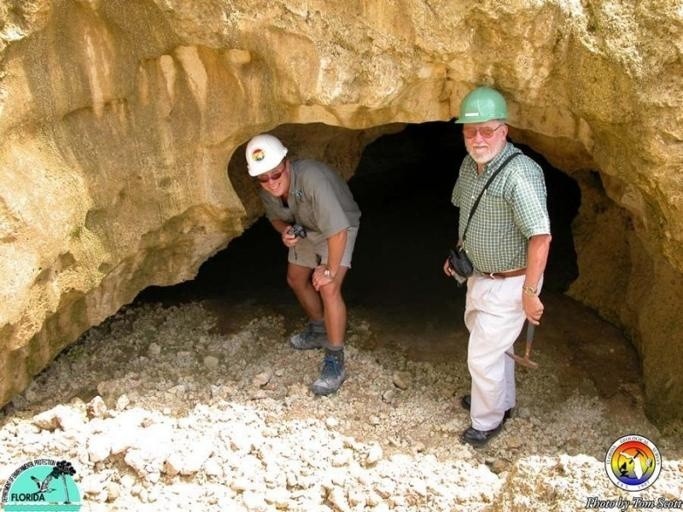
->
[481,269,527,279]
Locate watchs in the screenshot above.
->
[321,266,336,282]
[520,283,540,298]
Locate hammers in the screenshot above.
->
[505,323,539,369]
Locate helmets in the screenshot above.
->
[245,133,288,176]
[454,87,507,123]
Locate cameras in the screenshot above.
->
[287,224,306,239]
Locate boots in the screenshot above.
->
[289,318,329,349]
[312,344,345,395]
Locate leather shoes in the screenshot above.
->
[459,393,514,446]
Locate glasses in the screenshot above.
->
[257,164,287,182]
[463,124,503,140]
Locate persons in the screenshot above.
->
[245,134,362,396]
[443,88,553,445]
